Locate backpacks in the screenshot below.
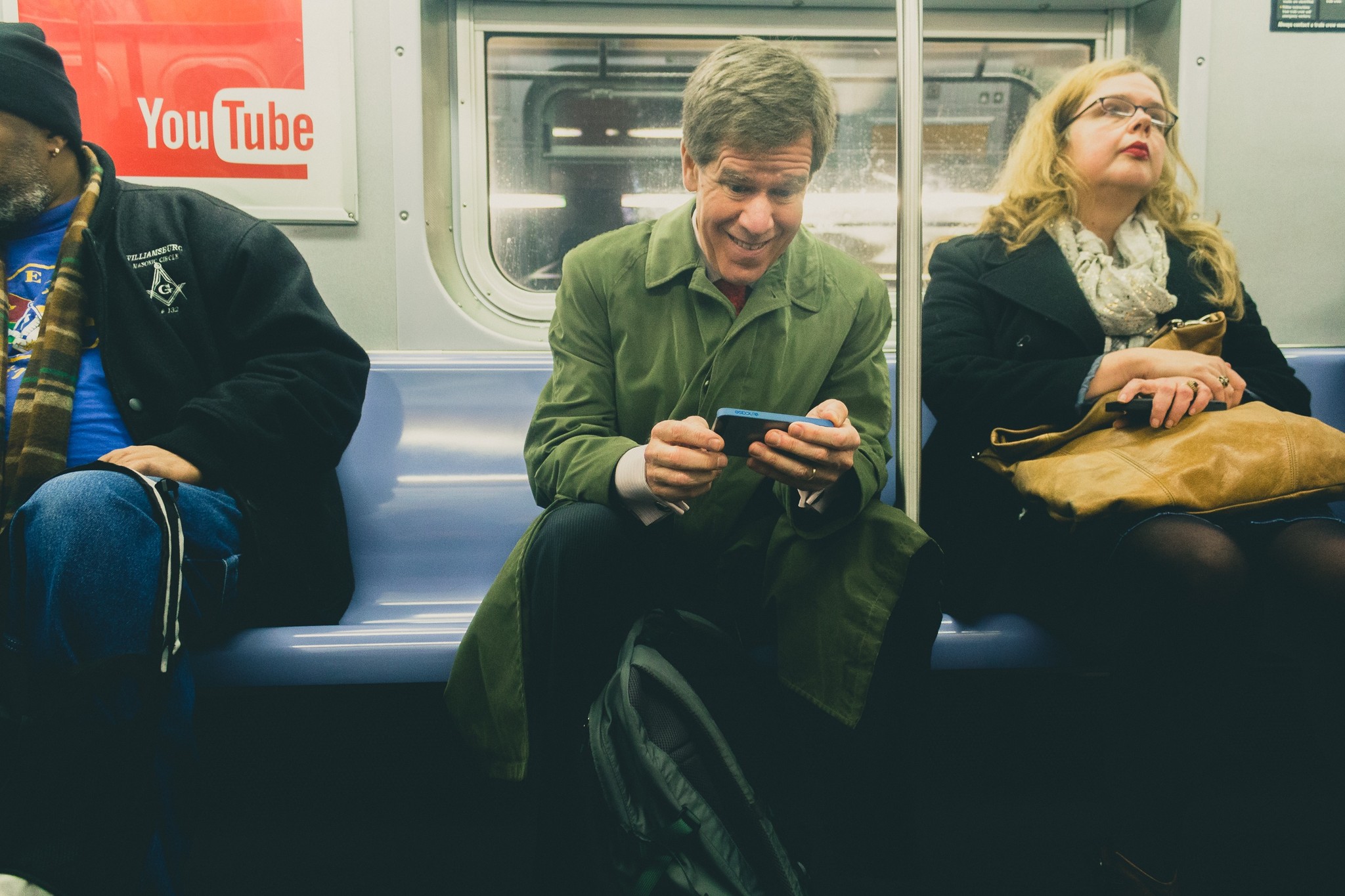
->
[588,602,814,896]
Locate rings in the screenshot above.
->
[1218,376,1230,389]
[1180,379,1199,393]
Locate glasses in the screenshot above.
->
[1058,97,1180,135]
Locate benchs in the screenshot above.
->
[202,345,1345,686]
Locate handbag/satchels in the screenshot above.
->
[976,310,1345,521]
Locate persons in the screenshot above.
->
[438,34,949,881]
[921,53,1344,853]
[0,19,374,896]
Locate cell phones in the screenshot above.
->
[706,409,836,459]
[1104,399,1228,413]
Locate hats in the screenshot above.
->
[1,23,81,152]
